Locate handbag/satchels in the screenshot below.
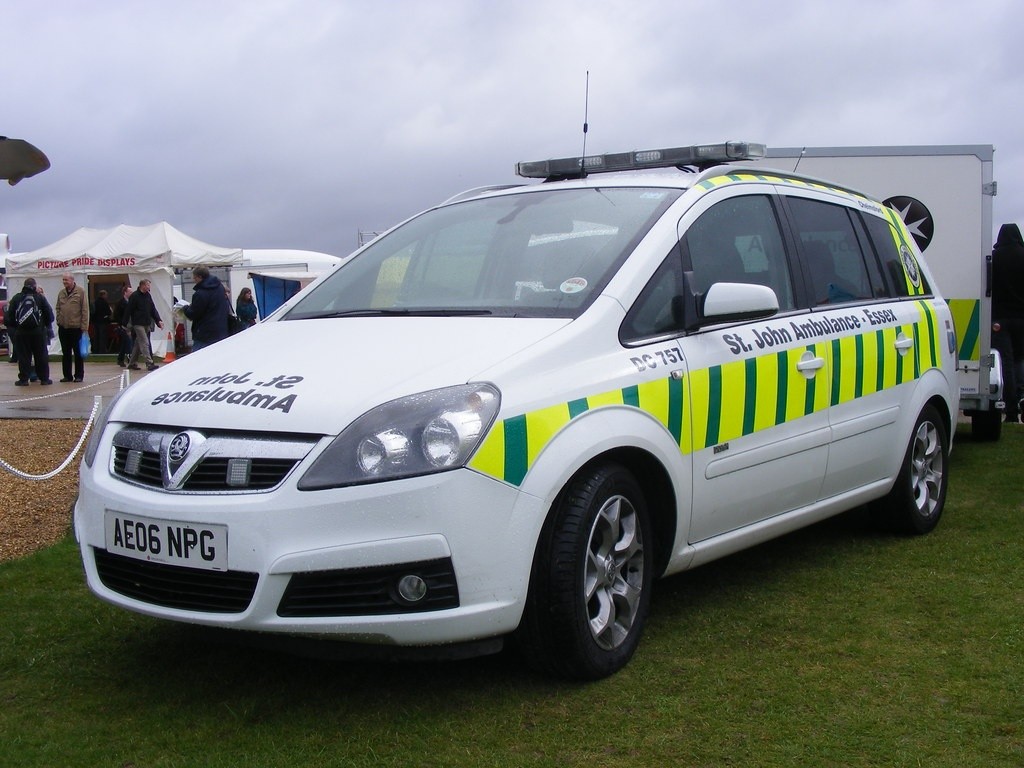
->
[224,294,245,336]
[79,331,91,360]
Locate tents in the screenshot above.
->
[2,221,249,356]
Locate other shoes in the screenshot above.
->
[147,365,159,370]
[127,365,141,370]
[118,361,126,367]
[41,379,52,385]
[15,379,29,386]
[73,377,82,382]
[60,377,73,382]
[31,376,37,382]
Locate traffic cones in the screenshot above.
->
[163,331,176,364]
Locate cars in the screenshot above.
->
[73,142,963,684]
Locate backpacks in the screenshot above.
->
[15,293,42,330]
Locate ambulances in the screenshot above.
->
[719,143,1006,444]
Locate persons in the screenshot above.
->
[6,268,259,387]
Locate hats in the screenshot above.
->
[224,287,231,292]
